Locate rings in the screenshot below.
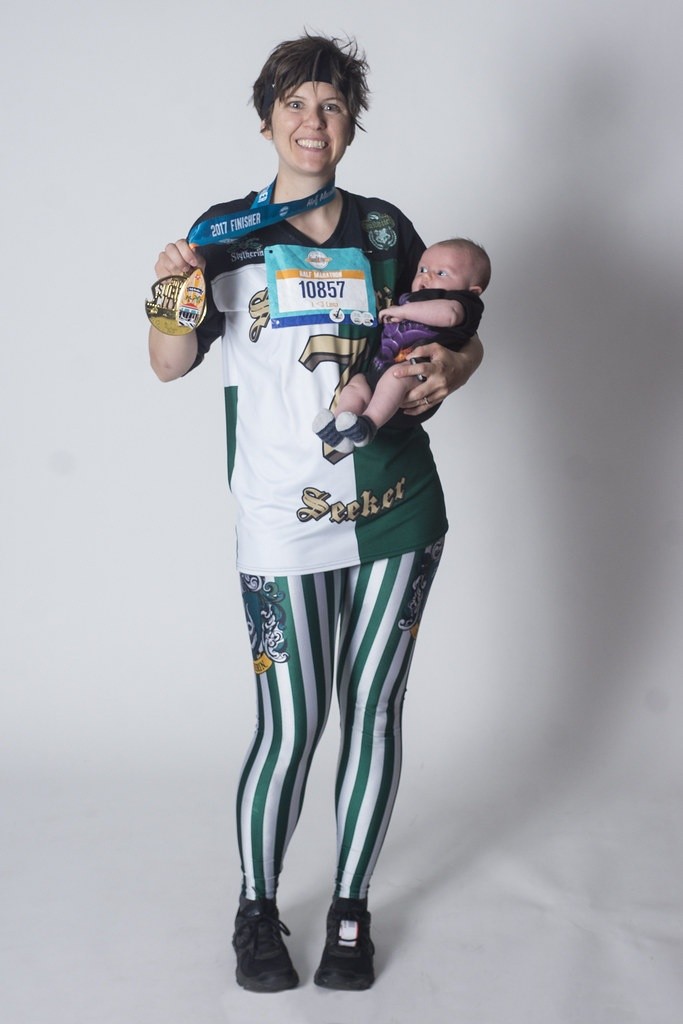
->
[423,397,429,405]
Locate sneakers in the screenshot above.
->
[232,894,300,993]
[314,895,375,990]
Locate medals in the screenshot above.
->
[145,275,208,336]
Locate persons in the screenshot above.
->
[147,36,485,993]
[311,237,491,455]
